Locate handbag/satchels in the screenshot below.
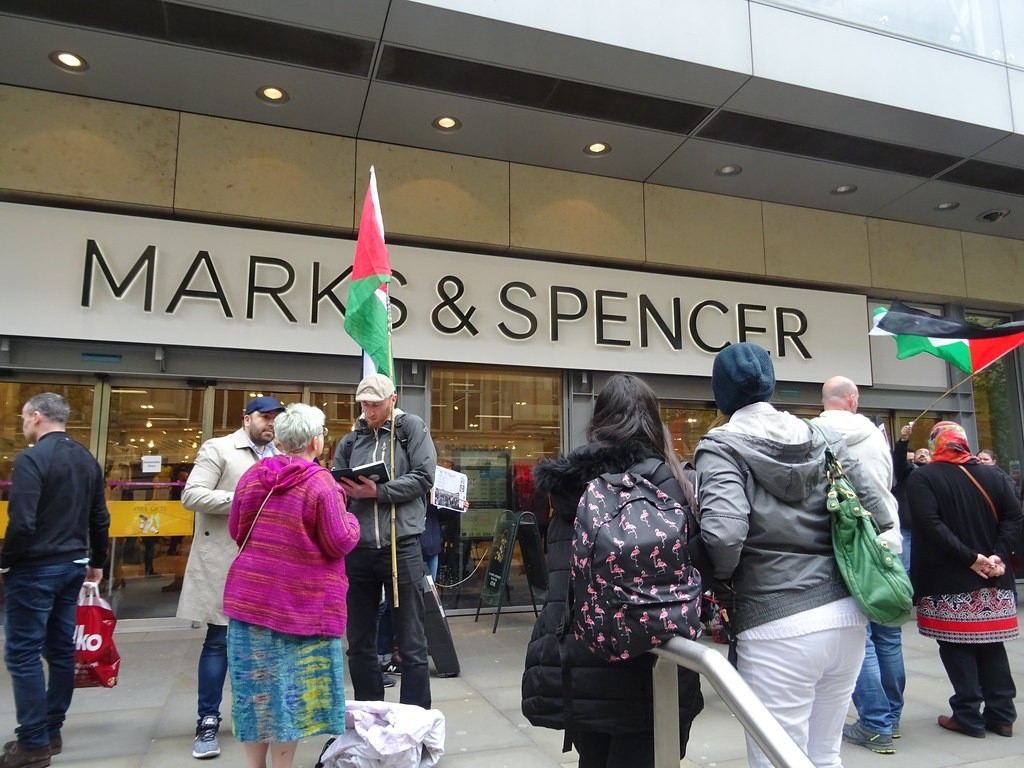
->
[803,417,914,628]
[72,582,120,690]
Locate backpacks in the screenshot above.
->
[555,458,702,670]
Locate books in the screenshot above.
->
[332,460,389,485]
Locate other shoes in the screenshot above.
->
[938,715,985,738]
[983,714,1012,736]
[161,578,183,591]
[5,734,62,756]
[146,572,159,575]
[0,744,51,768]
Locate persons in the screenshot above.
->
[907,420,1024,739]
[153,455,173,500]
[331,374,437,708]
[435,488,459,509]
[693,343,895,768]
[0,393,111,768]
[106,430,137,481]
[99,462,195,592]
[976,449,998,465]
[811,376,907,752]
[375,493,470,687]
[892,426,931,575]
[174,396,287,757]
[907,450,915,464]
[222,403,360,768]
[522,374,715,768]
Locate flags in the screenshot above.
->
[870,296,1024,376]
[343,166,396,390]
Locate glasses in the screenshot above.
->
[315,426,328,437]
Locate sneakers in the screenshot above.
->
[192,715,222,757]
[892,723,901,738]
[842,720,895,753]
[382,675,396,688]
[381,662,402,675]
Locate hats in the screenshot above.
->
[712,342,775,414]
[245,397,286,415]
[356,373,395,402]
